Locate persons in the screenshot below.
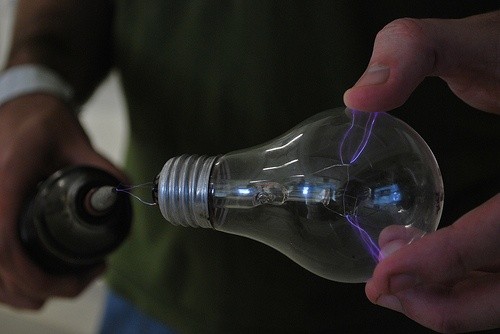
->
[0,0,500,334]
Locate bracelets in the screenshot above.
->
[0,62,70,105]
